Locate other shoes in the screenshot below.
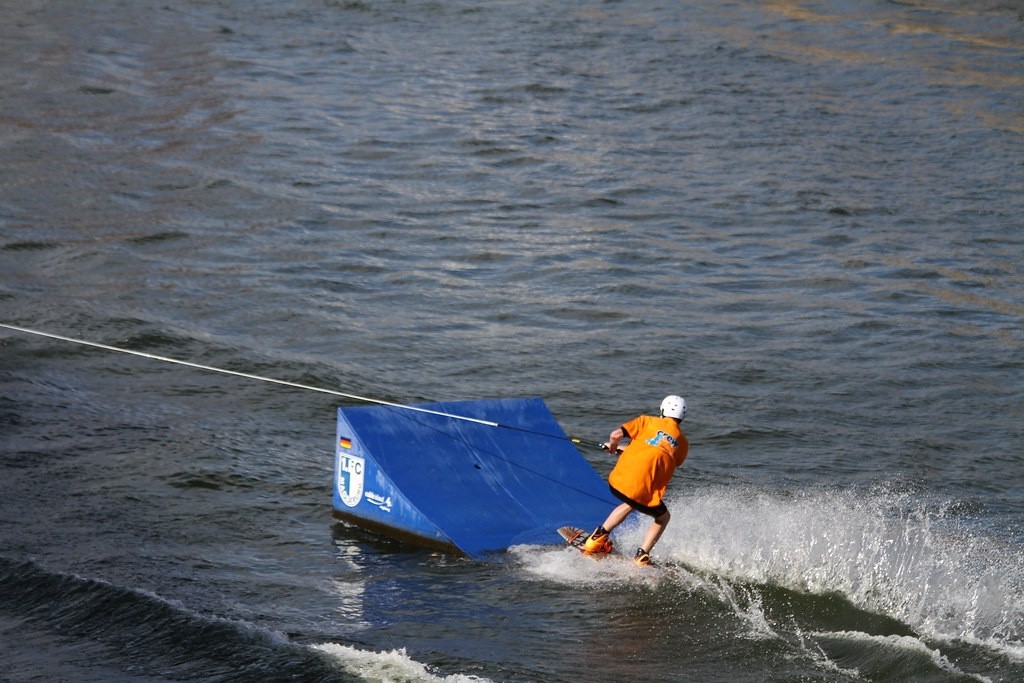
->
[586,526,613,553]
[634,548,655,565]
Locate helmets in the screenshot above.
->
[660,395,687,422]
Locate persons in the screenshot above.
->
[585,394,689,566]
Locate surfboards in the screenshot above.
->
[556,526,624,560]
